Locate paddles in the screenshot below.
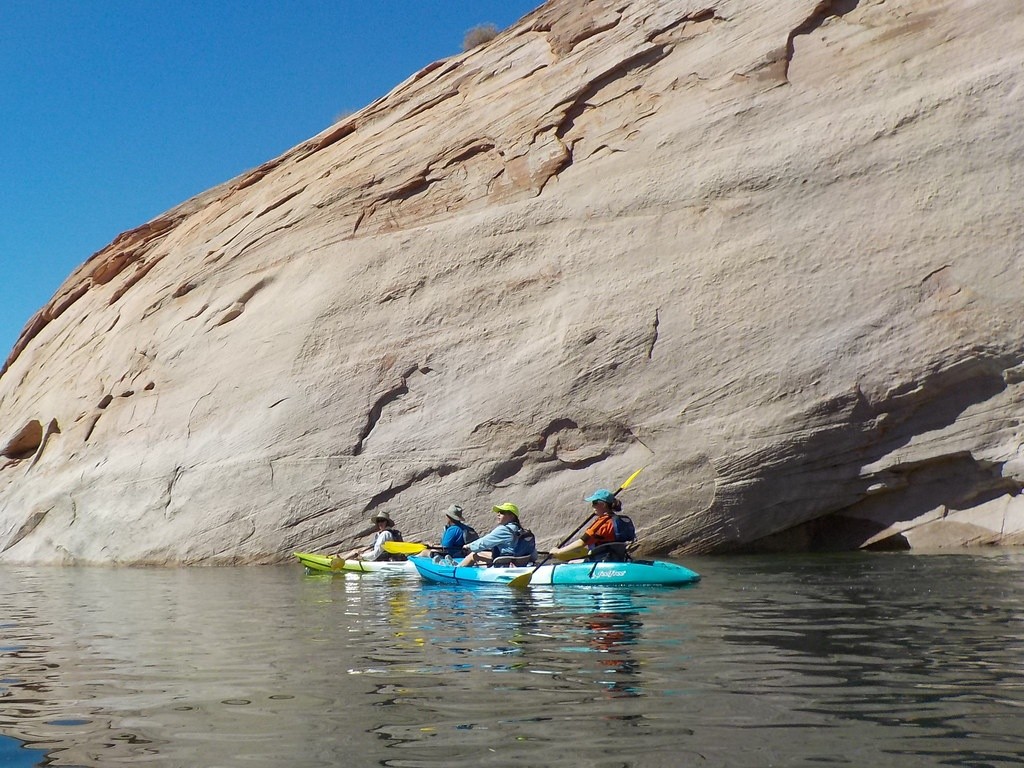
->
[506,466,644,588]
[382,540,550,555]
[330,545,374,574]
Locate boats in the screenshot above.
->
[293,552,417,572]
[408,556,702,585]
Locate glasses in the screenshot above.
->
[376,519,385,523]
[497,510,505,514]
[593,501,602,506]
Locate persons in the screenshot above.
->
[337,511,406,561]
[432,502,538,567]
[414,505,479,558]
[509,488,626,567]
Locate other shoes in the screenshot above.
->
[445,555,459,567]
[432,555,446,565]
[335,554,342,560]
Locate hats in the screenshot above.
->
[585,489,614,503]
[442,504,465,521]
[493,503,519,517]
[371,511,395,528]
